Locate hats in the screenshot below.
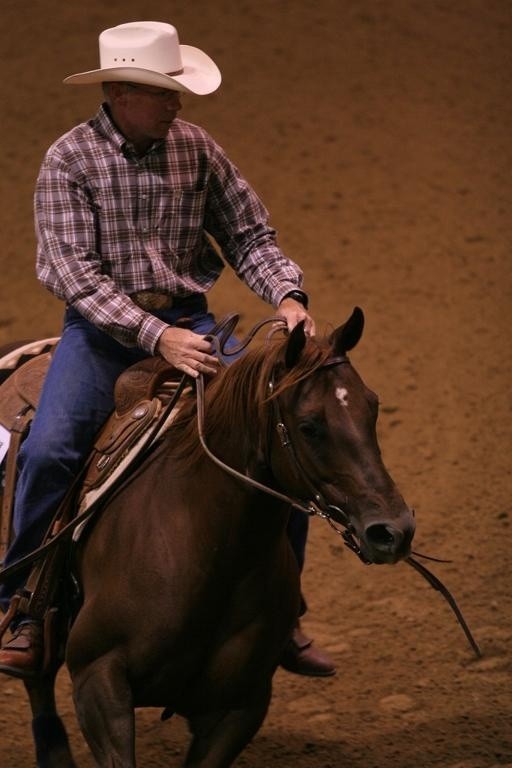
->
[62,21,222,96]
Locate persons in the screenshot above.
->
[0,21,317,676]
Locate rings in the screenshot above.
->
[304,328,310,335]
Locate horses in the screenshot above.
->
[0,306,416,768]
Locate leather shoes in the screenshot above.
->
[0,618,44,680]
